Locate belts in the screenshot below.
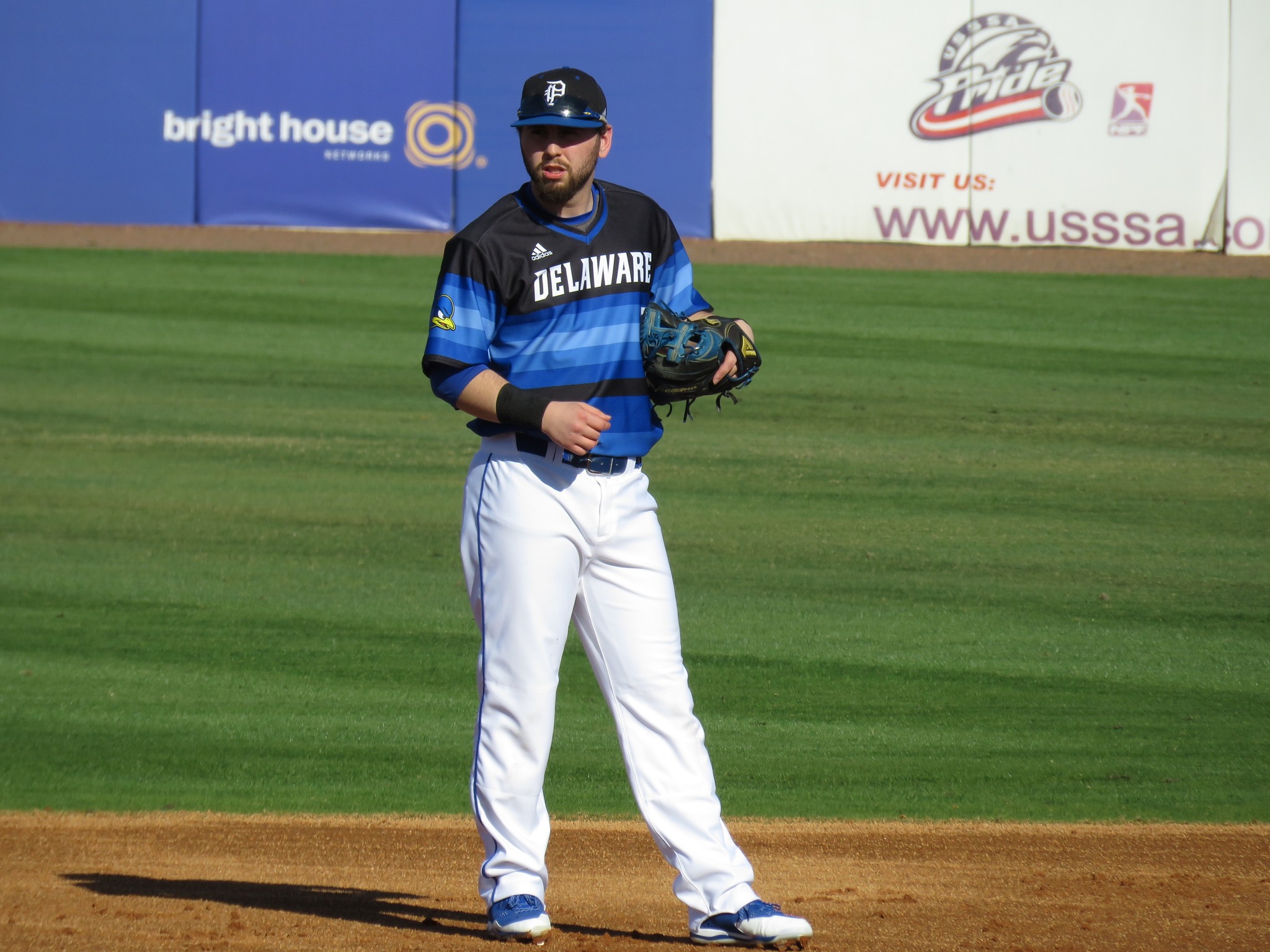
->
[515,434,640,473]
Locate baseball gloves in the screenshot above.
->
[640,301,762,406]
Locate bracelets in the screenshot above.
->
[495,382,551,431]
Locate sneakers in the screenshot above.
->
[690,899,814,945]
[487,894,552,938]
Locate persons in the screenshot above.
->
[422,67,815,947]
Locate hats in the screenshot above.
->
[510,65,607,127]
